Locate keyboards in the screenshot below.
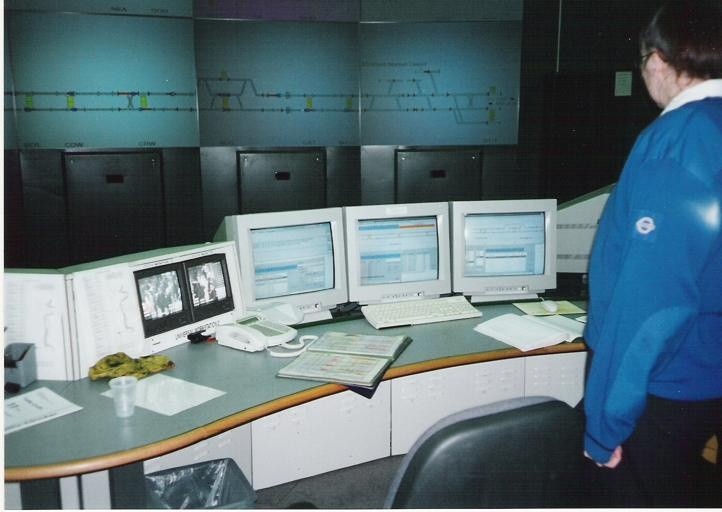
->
[362,296,483,329]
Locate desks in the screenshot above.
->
[5,299,587,510]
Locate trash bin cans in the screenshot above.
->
[144,458,258,509]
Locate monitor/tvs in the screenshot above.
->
[344,203,450,304]
[214,207,343,325]
[449,198,557,304]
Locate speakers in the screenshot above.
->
[394,144,483,202]
[236,149,327,211]
[62,146,164,258]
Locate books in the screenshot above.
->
[475,315,588,352]
[276,330,412,400]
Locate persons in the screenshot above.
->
[582,1,721,505]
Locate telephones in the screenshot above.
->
[215,314,298,353]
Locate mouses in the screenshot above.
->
[540,300,559,313]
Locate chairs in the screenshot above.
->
[384,395,585,509]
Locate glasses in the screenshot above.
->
[631,52,660,75]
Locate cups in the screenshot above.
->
[107,375,138,419]
